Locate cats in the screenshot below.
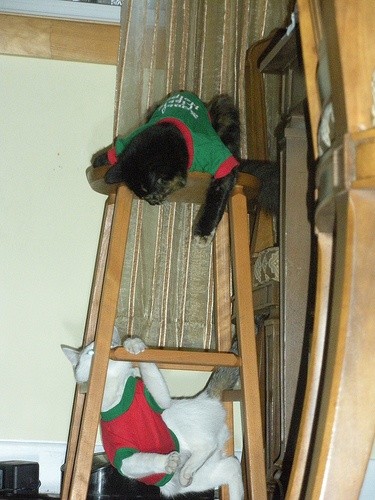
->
[61,307,271,500]
[92,91,281,248]
